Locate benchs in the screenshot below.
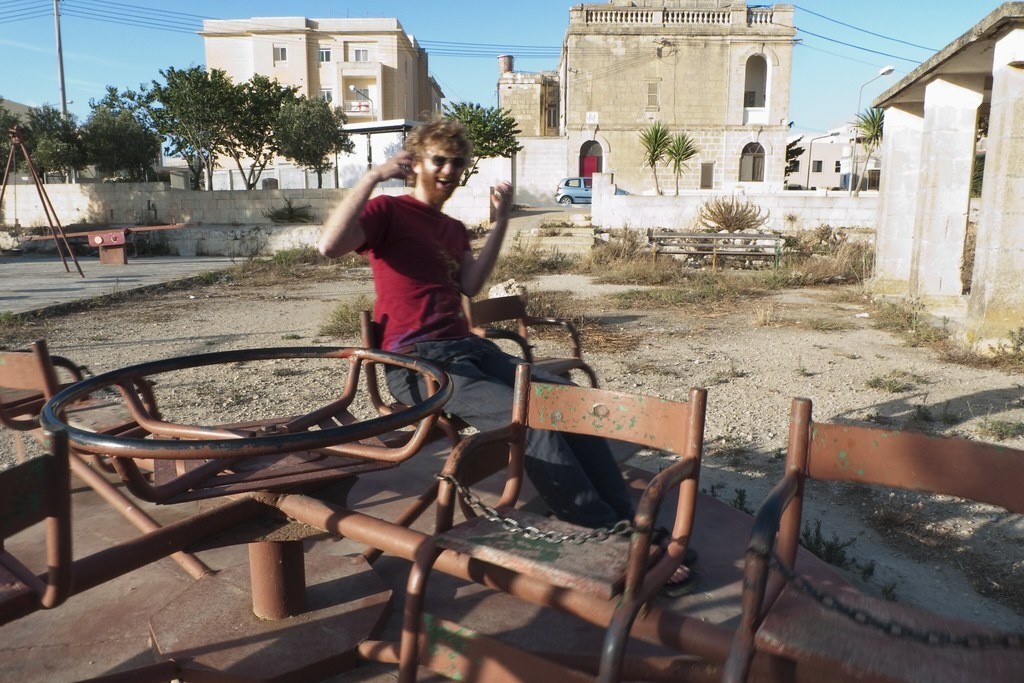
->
[646,227,781,272]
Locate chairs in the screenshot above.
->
[396,362,708,683]
[0,340,162,459]
[723,398,1024,683]
[360,285,600,453]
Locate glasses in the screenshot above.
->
[413,154,466,169]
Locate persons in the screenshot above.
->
[318,118,698,585]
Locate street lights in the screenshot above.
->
[350,85,374,122]
[807,132,840,190]
[847,67,895,196]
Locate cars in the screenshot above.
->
[556,176,629,204]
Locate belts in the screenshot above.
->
[394,345,417,355]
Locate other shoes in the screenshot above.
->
[682,548,698,564]
[660,565,700,597]
